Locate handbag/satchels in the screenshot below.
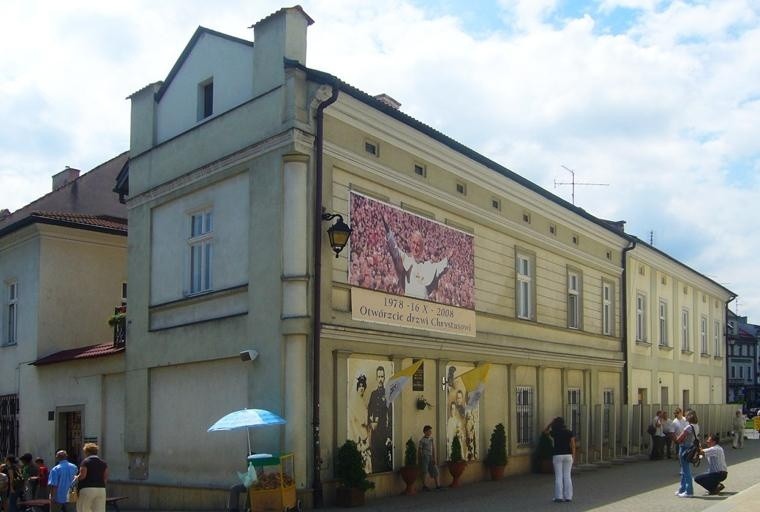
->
[66,486,78,503]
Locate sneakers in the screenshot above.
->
[674,490,682,495]
[678,492,693,498]
[705,491,720,496]
[553,498,572,502]
[716,484,724,492]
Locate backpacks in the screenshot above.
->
[682,424,700,467]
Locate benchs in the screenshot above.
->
[62,494,127,511]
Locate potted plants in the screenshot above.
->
[400,435,421,497]
[447,431,469,488]
[534,436,554,475]
[334,438,379,506]
[483,422,512,482]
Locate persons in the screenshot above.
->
[351,367,395,471]
[229,451,262,512]
[0,442,109,512]
[449,366,476,460]
[350,192,475,311]
[545,418,579,505]
[418,426,443,491]
[646,408,746,499]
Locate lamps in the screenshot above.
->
[321,208,352,257]
[238,351,257,364]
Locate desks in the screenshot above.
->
[14,498,53,511]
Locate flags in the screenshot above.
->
[387,360,424,407]
[462,363,492,392]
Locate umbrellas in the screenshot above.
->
[209,408,286,458]
